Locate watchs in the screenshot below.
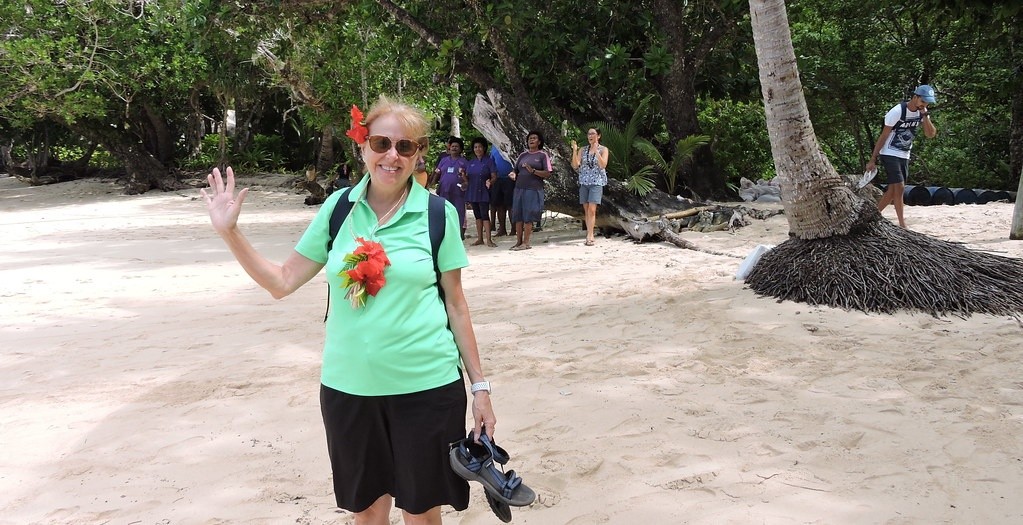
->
[470,381,492,396]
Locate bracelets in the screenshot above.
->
[531,168,536,175]
[921,111,929,118]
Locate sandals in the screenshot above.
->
[450,434,535,507]
[468,423,512,523]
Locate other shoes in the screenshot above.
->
[584,236,595,246]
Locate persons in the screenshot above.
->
[571,127,609,246]
[865,85,937,231]
[332,131,553,252]
[201,100,497,525]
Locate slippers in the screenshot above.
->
[510,243,532,250]
[859,168,878,188]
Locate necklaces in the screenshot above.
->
[587,144,595,168]
[348,177,409,250]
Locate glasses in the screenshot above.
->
[587,133,598,136]
[365,135,422,158]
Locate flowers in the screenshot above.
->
[346,104,368,143]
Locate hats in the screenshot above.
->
[914,85,935,102]
